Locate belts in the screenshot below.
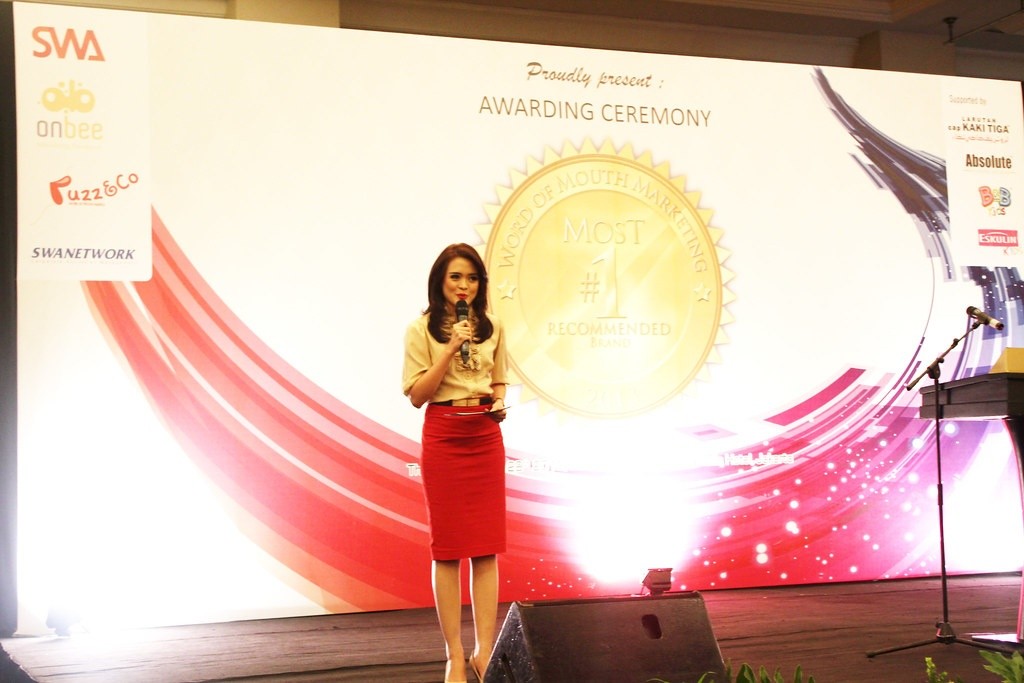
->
[430,395,493,406]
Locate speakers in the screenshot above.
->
[484,592,733,683]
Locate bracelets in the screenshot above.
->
[492,397,505,407]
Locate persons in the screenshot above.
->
[402,243,510,683]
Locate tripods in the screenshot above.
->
[866,317,1024,659]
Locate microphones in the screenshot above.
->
[966,306,1004,330]
[455,300,469,364]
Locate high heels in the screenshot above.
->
[468,651,483,683]
